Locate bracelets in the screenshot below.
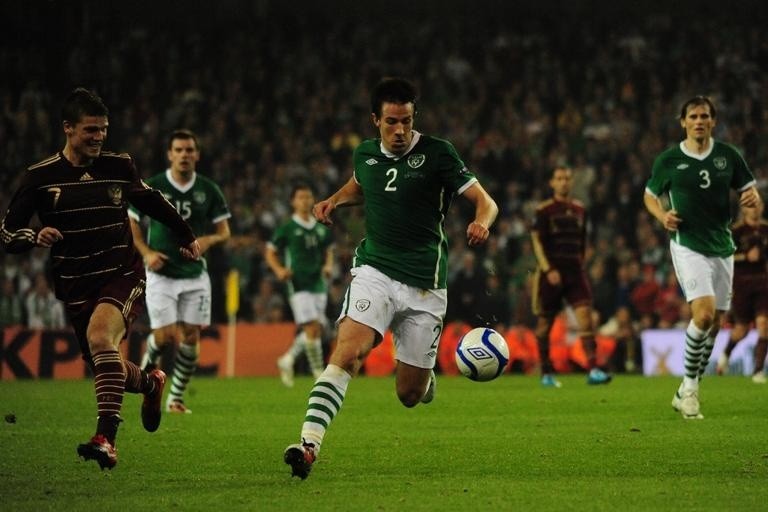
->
[542,265,555,276]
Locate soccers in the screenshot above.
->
[453,327,510,381]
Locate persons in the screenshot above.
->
[263,185,336,389]
[527,165,613,389]
[416,34,687,165]
[643,96,760,421]
[282,77,500,480]
[462,166,550,330]
[126,130,232,415]
[145,67,356,181]
[715,195,768,384]
[226,198,280,323]
[3,252,63,328]
[593,166,678,371]
[0,88,201,470]
[569,155,595,231]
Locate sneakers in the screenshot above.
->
[420,369,437,404]
[283,437,317,482]
[540,372,563,387]
[164,400,193,414]
[587,366,613,385]
[77,433,118,472]
[680,388,704,419]
[140,369,167,433]
[670,390,682,411]
[276,355,295,388]
